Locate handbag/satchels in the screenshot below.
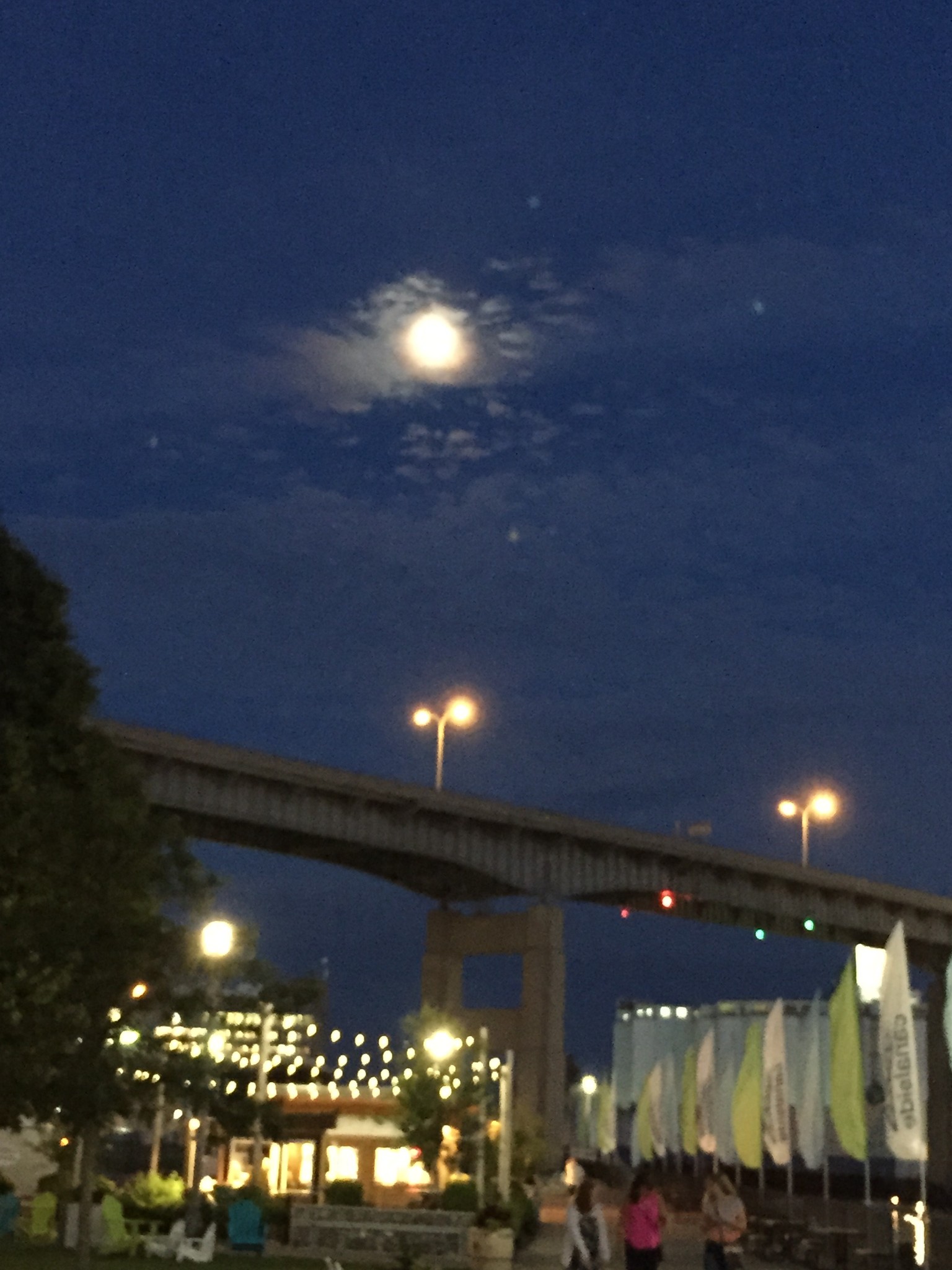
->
[722,1243,746,1270]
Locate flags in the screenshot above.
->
[567,920,930,1166]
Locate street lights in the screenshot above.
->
[410,692,481,787]
[778,786,841,865]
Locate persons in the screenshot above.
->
[695,1170,749,1269]
[614,1170,669,1270]
[556,1175,609,1269]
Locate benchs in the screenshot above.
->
[288,1201,473,1252]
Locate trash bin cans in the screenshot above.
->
[465,1209,515,1270]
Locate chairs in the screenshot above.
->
[17,1180,270,1262]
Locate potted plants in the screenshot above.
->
[467,1203,516,1260]
